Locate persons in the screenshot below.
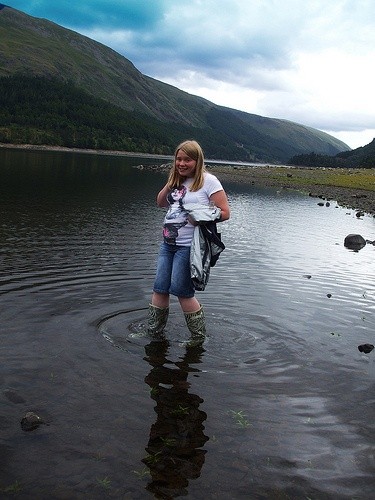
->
[147,140,231,336]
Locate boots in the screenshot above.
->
[127,302,169,342]
[178,304,206,347]
